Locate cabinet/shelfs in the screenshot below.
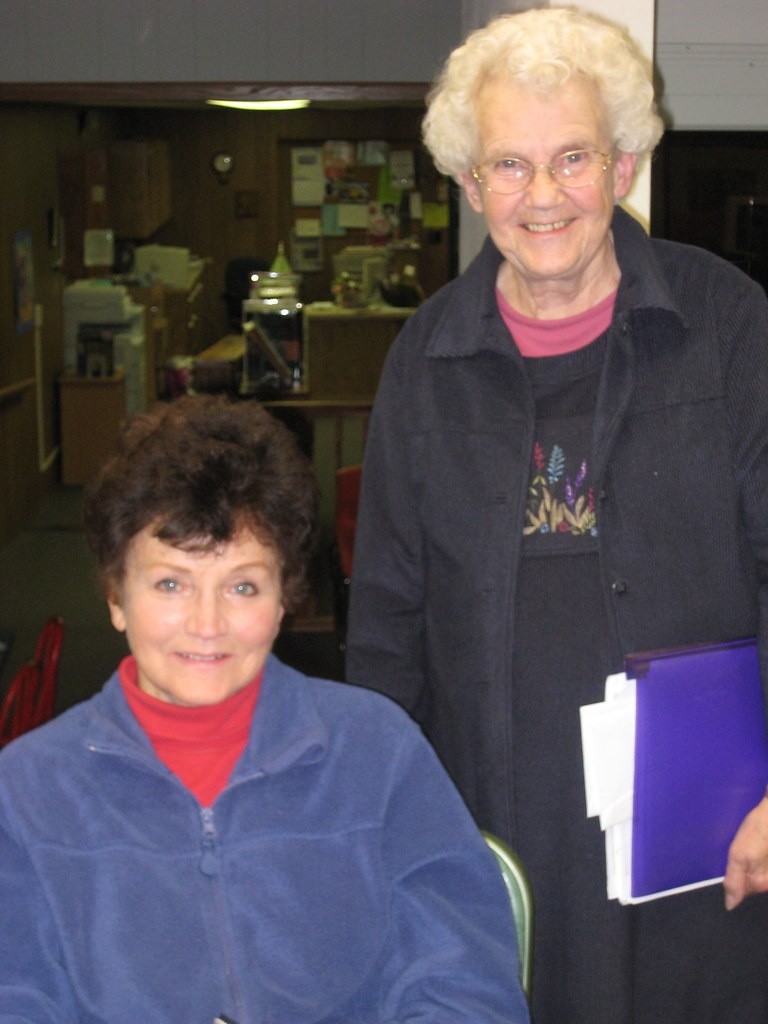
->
[58,274,418,489]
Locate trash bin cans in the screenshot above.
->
[58,365,128,487]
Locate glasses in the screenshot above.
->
[466,140,619,194]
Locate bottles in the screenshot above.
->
[83,224,114,267]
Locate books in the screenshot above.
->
[580,635,768,904]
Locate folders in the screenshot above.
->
[622,633,766,899]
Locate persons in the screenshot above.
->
[0,397,538,1024]
[346,8,768,1024]
